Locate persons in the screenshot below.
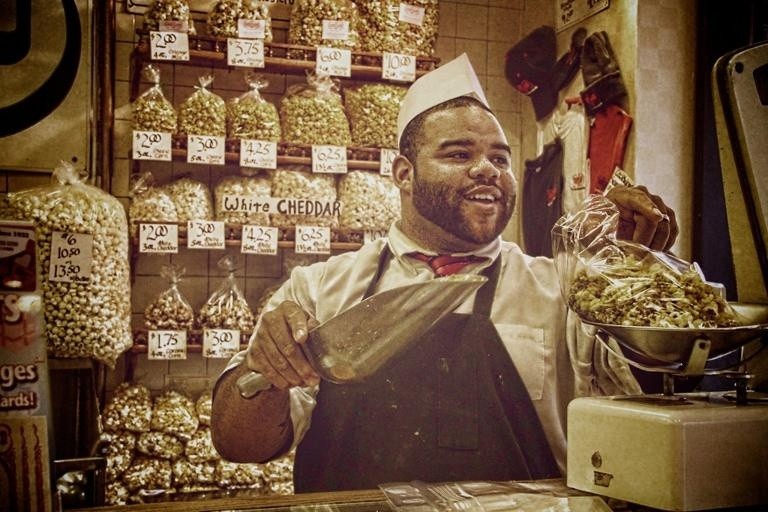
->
[213,50,678,493]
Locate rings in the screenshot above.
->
[659,214,670,223]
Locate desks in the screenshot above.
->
[72,478,617,512]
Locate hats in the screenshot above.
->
[503,25,559,122]
[397,52,495,156]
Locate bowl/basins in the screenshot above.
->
[582,299,768,361]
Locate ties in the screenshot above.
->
[407,253,490,277]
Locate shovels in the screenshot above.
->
[237,273,489,400]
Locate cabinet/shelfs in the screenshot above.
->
[43,360,108,511]
[121,28,439,401]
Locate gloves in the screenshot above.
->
[579,31,628,119]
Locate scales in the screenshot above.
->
[567,311,768,512]
